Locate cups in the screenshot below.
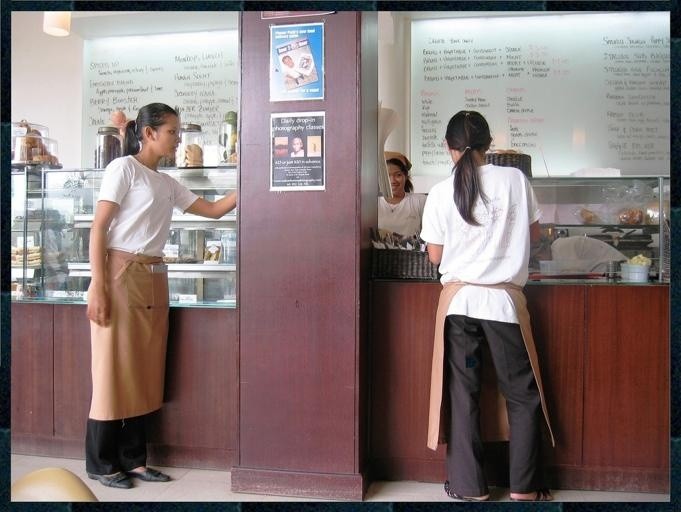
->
[620,264,651,282]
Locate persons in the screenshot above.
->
[83,101,237,489]
[378,151,428,239]
[290,137,304,157]
[421,109,556,502]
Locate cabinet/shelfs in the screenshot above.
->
[11,163,239,309]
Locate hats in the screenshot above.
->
[384,151,414,171]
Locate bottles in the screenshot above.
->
[175,123,204,168]
[94,125,124,170]
[218,110,239,167]
[219,232,236,265]
[157,154,176,168]
[16,276,38,299]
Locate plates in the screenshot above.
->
[296,53,314,76]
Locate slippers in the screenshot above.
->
[443,476,493,502]
[509,486,556,503]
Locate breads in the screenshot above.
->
[619,208,643,224]
[111,111,127,136]
[581,209,599,225]
[11,247,42,266]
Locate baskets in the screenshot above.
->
[484,153,534,183]
[367,243,441,283]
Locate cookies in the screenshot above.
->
[186,144,203,166]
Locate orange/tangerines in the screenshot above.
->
[225,110,237,124]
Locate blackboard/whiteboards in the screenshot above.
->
[78,29,239,168]
[409,10,672,179]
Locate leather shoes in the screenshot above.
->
[86,465,135,489]
[125,463,172,483]
